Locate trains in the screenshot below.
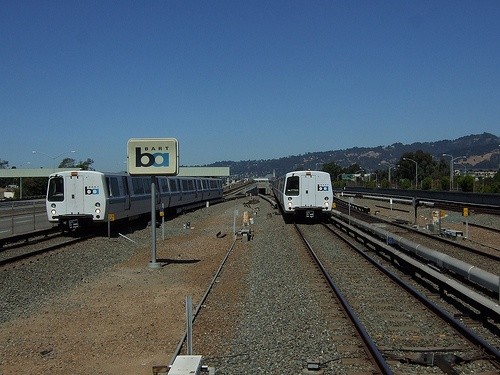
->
[46,170,222,234]
[272,171,334,221]
[0,168,82,240]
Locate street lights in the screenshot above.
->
[355,153,467,191]
[26,149,77,169]
